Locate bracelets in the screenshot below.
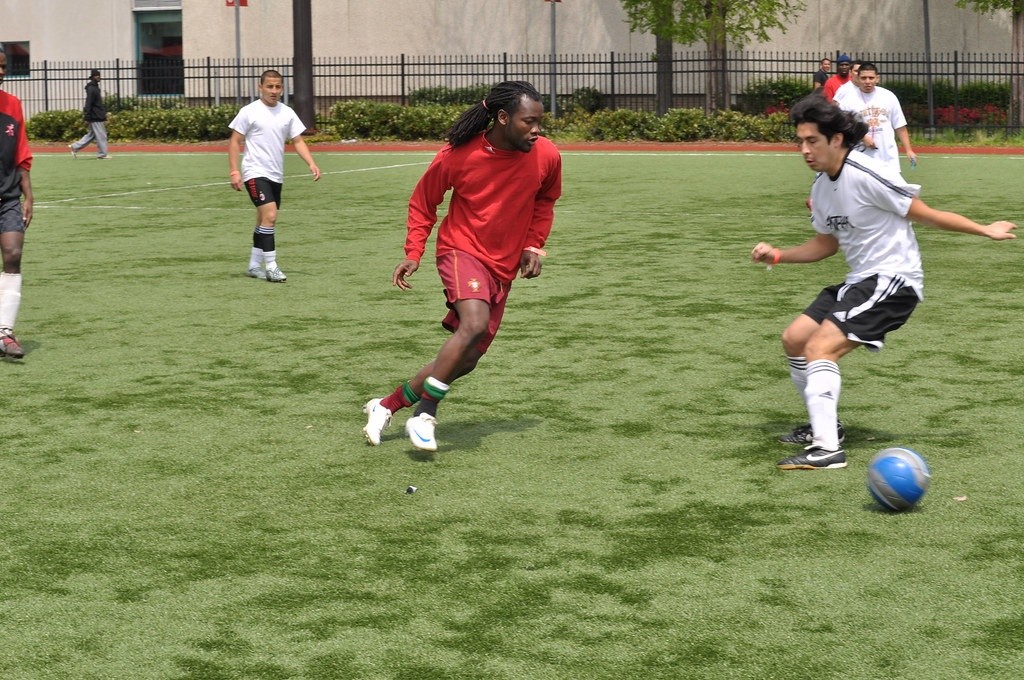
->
[773,248,780,263]
[230,171,239,176]
[523,247,547,257]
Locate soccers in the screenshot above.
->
[866,447,931,511]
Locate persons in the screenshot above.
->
[70,69,113,159]
[837,62,919,173]
[361,81,563,453]
[820,54,852,103]
[750,90,1018,470]
[227,70,320,282]
[0,43,33,356]
[832,60,863,107]
[814,57,831,90]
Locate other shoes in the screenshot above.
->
[98,154,112,160]
[69,144,77,159]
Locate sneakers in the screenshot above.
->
[774,442,848,471]
[0,329,25,359]
[247,265,267,279]
[264,265,286,283]
[777,420,845,445]
[361,398,391,445]
[406,412,438,452]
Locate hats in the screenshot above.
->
[89,70,100,81]
[836,55,850,64]
